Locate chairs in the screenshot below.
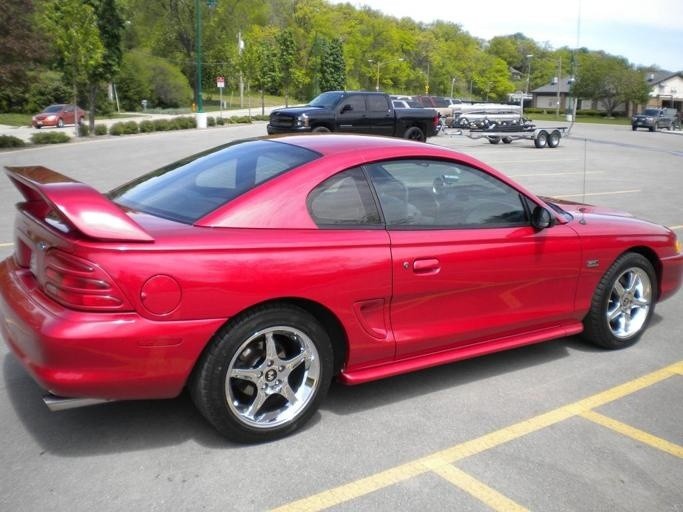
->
[335,180,424,228]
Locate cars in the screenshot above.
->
[31,104,86,128]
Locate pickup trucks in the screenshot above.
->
[630,106,674,132]
[268,91,442,149]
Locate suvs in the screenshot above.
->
[386,92,461,112]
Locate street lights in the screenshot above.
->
[419,71,429,96]
[450,77,493,102]
[525,52,533,94]
[366,56,404,93]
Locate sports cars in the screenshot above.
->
[4,135,683,448]
